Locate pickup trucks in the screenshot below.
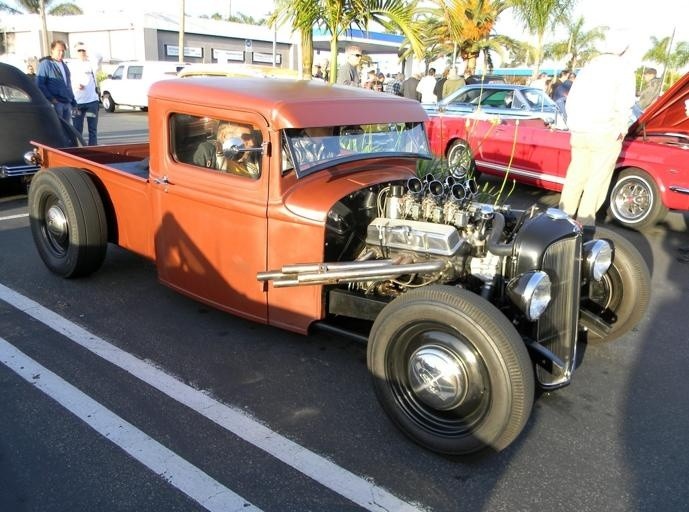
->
[25,79,652,463]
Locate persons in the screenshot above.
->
[636,68,662,111]
[313,45,577,108]
[26,40,101,145]
[216,123,259,178]
[559,41,636,226]
[282,128,337,172]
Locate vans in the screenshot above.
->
[99,61,300,112]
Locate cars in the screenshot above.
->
[421,72,689,230]
[1,61,90,201]
